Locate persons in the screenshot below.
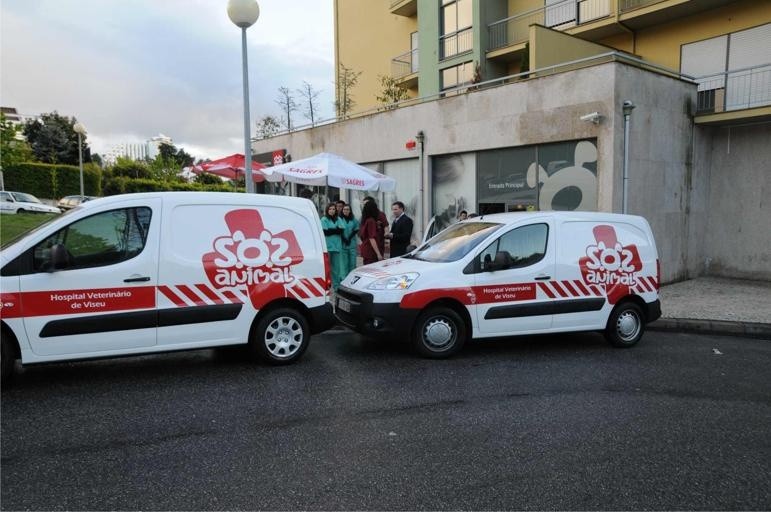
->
[461,210,467,220]
[468,214,478,219]
[321,196,413,292]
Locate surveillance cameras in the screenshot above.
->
[581,111,600,124]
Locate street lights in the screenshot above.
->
[69,122,90,201]
[227,0,260,196]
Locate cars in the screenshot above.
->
[58,196,97,212]
[0,191,62,217]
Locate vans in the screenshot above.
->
[0,192,336,380]
[335,209,662,358]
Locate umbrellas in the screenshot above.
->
[259,152,395,216]
[191,154,269,192]
